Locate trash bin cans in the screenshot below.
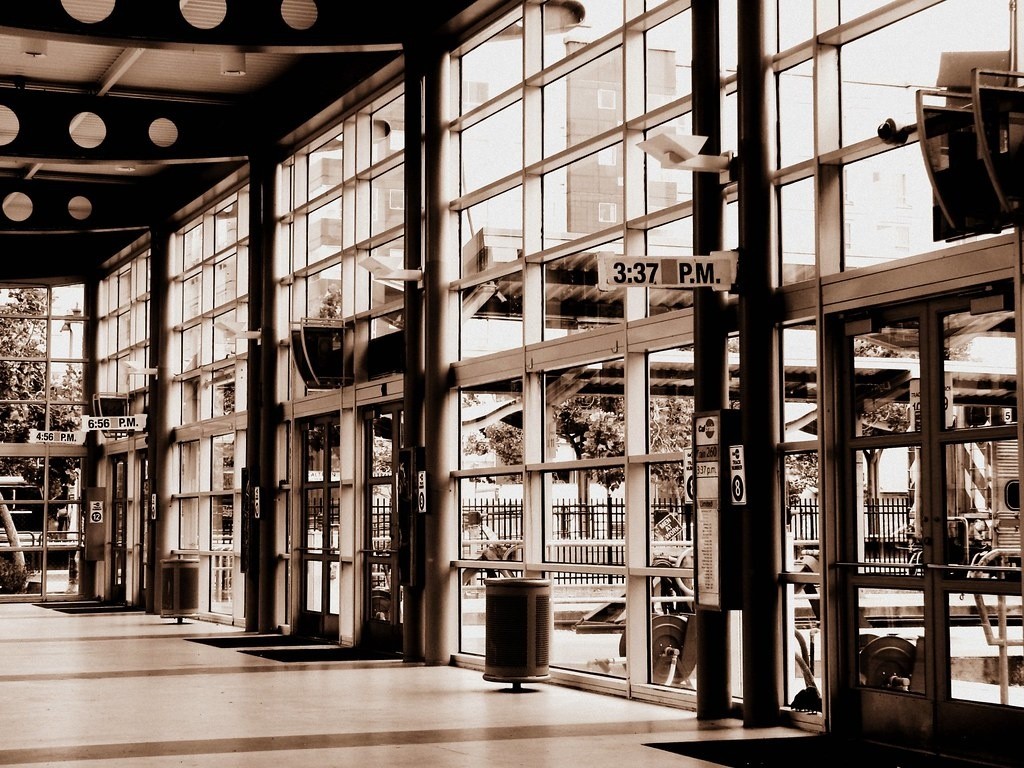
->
[482,577,552,684]
[159,559,201,617]
